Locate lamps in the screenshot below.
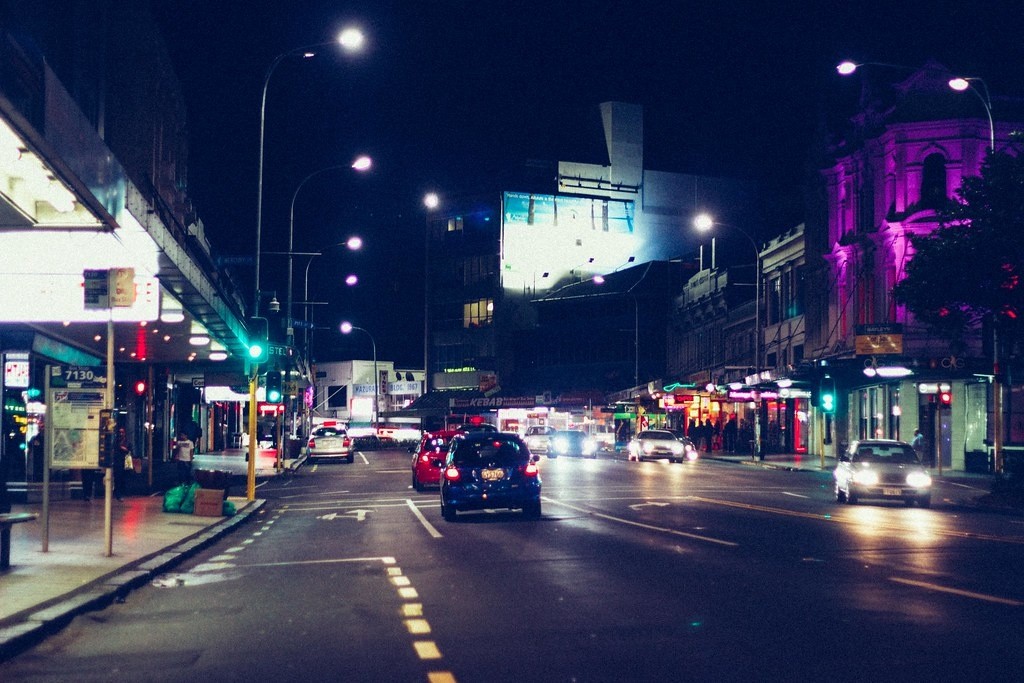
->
[569,257,594,274]
[616,256,635,272]
[528,273,549,289]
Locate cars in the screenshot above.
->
[521,424,557,455]
[431,430,543,522]
[667,430,699,461]
[832,438,933,509]
[453,422,500,436]
[305,425,355,465]
[626,429,686,464]
[410,430,468,493]
[546,429,597,459]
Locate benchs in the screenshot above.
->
[0,512,39,573]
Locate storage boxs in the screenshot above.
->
[194,489,225,517]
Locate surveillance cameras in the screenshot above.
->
[268,302,280,314]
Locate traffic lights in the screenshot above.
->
[265,370,283,405]
[819,375,836,414]
[246,317,269,364]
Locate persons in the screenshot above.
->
[28,422,132,501]
[915,430,932,468]
[687,417,749,452]
[177,432,194,485]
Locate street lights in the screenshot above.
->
[340,321,380,451]
[282,155,372,465]
[300,236,363,450]
[593,275,639,415]
[836,58,1011,498]
[243,25,366,504]
[694,214,760,392]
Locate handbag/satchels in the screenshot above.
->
[125,451,133,471]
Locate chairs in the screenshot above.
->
[859,449,867,459]
[496,442,516,461]
[456,444,481,461]
[892,453,904,457]
